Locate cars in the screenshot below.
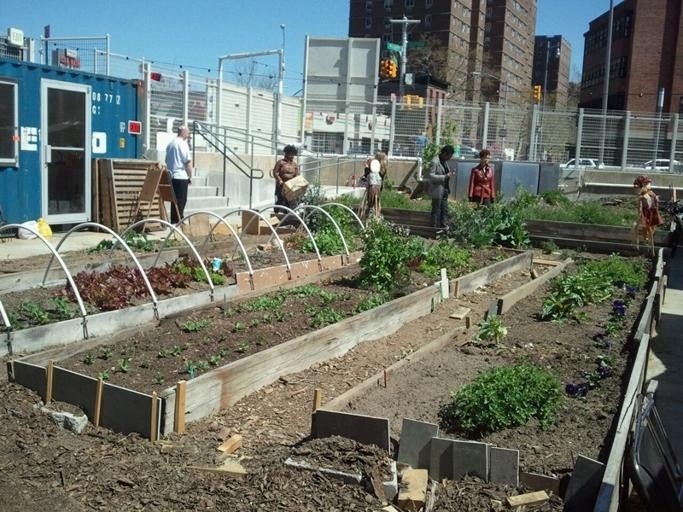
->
[560,158,605,169]
[459,146,479,157]
[645,159,683,170]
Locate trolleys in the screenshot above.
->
[657,200,683,258]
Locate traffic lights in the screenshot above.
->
[533,85,542,102]
[380,59,398,79]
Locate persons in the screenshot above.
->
[630,175,664,252]
[361,152,388,222]
[163,125,195,225]
[364,159,383,214]
[271,144,299,211]
[468,149,496,211]
[428,145,457,237]
[390,127,427,158]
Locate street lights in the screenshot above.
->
[281,23,286,82]
[543,40,560,106]
